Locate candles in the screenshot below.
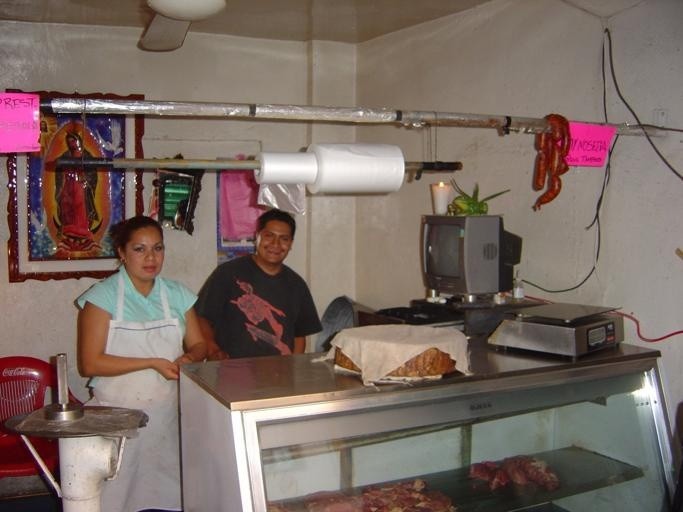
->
[430,181,451,216]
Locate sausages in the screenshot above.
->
[532,114,571,212]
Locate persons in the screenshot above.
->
[50,132,103,250]
[183,209,323,361]
[39,121,49,152]
[75,216,208,511]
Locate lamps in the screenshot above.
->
[145,1,226,22]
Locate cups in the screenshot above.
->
[431,184,450,215]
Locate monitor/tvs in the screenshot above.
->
[420,213,522,308]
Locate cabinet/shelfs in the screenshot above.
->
[176,334,679,511]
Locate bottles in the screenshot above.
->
[513,270,524,299]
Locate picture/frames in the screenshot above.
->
[3,88,145,284]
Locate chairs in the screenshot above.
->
[0,355,83,480]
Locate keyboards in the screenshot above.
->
[378,306,436,319]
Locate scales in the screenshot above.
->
[485,303,625,363]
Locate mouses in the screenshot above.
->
[410,310,431,320]
